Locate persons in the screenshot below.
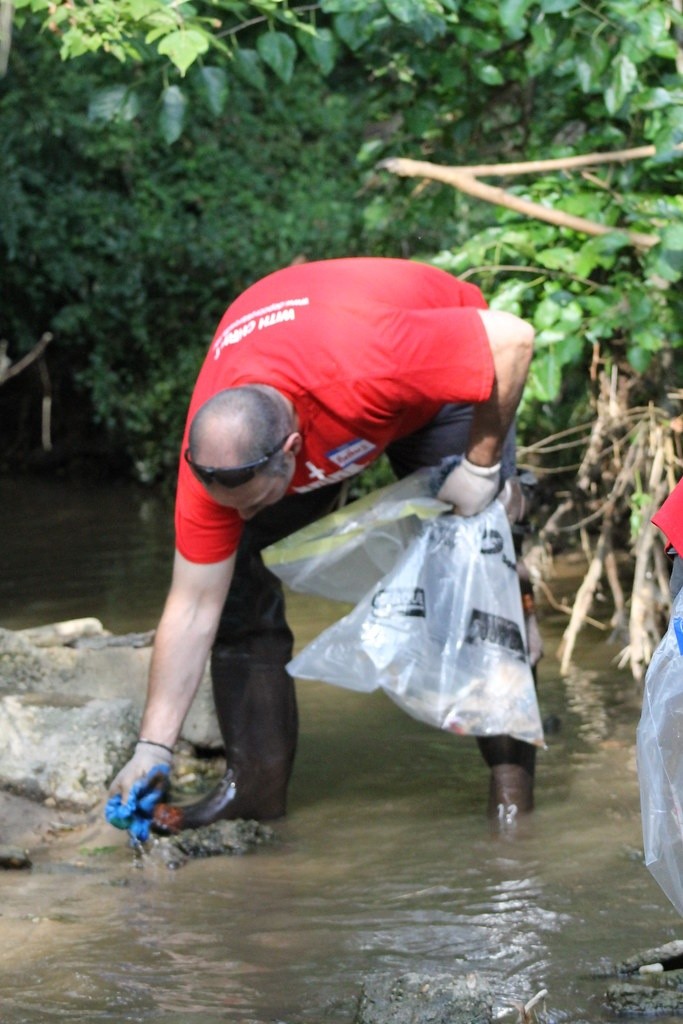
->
[106,258,538,841]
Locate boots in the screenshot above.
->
[148,648,299,835]
[475,736,536,818]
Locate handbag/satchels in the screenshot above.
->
[259,457,549,756]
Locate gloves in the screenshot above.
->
[437,452,504,518]
[102,742,173,842]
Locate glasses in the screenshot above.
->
[184,435,290,489]
